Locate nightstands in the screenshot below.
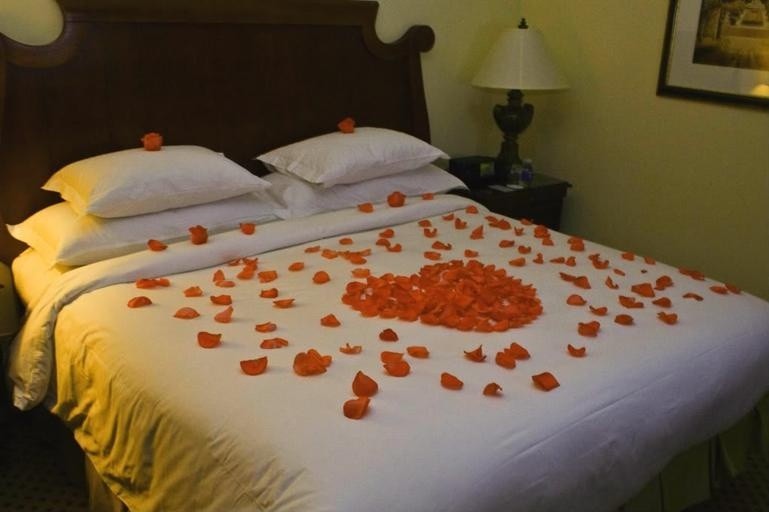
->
[446,171,573,231]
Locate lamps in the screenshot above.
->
[471,14,572,188]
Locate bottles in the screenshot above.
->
[520,159,534,185]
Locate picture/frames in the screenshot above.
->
[654,0,768,108]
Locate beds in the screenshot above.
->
[1,2,769,511]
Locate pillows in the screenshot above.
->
[41,145,273,219]
[255,127,452,190]
[255,163,471,220]
[7,192,279,273]
